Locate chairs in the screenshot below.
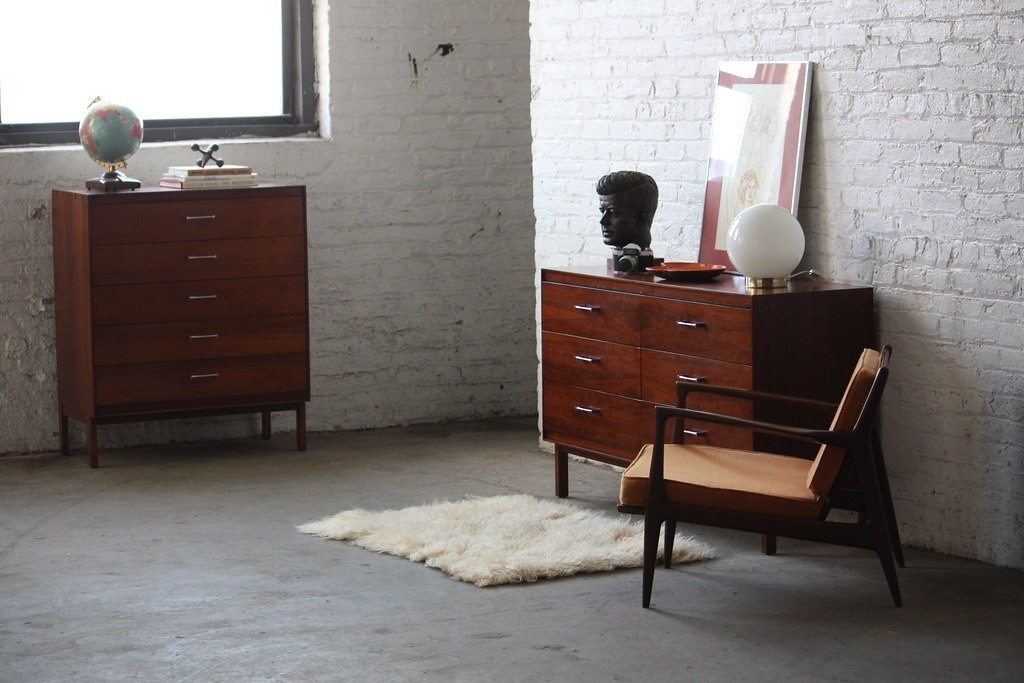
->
[615,345,909,610]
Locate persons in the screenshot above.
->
[597,172,661,250]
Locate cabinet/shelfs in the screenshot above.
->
[48,183,312,469]
[540,264,874,554]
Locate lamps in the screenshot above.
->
[727,202,805,290]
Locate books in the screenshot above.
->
[159,166,258,187]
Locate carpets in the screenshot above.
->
[293,491,721,589]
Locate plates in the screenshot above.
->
[645,262,726,282]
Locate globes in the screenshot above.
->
[80,96,144,193]
